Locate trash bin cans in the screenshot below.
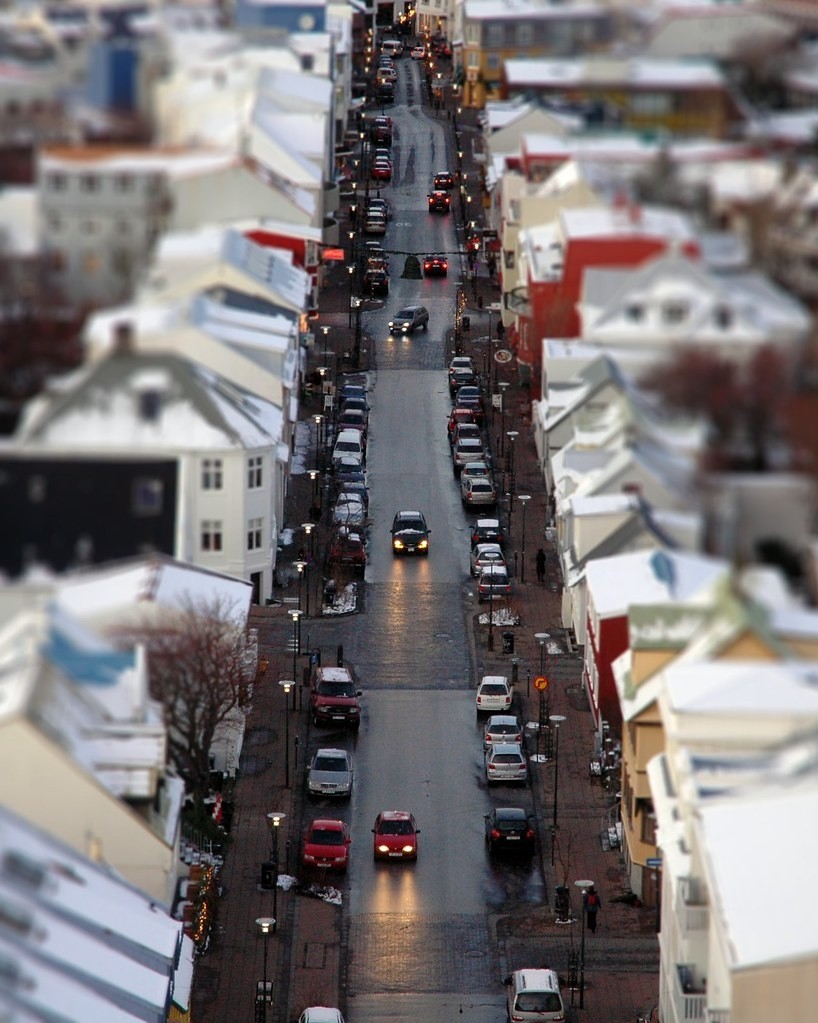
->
[502,632,515,654]
[324,592,334,604]
[554,885,570,921]
[303,666,310,687]
[261,861,275,890]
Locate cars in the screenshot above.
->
[328,384,372,579]
[372,810,420,865]
[363,37,459,335]
[303,820,352,877]
[445,354,529,787]
[389,511,431,555]
[298,1007,345,1023]
[306,749,354,800]
[482,807,535,854]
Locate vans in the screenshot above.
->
[309,667,362,731]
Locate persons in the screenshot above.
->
[488,257,496,279]
[536,549,546,583]
[583,886,601,933]
[496,318,505,341]
[468,253,476,270]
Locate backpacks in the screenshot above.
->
[587,893,599,906]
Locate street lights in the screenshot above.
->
[574,880,594,1009]
[288,610,303,711]
[292,558,308,655]
[301,523,316,617]
[495,381,566,827]
[279,681,296,789]
[266,812,286,934]
[255,918,276,1023]
[305,101,367,556]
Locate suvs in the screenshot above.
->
[503,968,566,1023]
